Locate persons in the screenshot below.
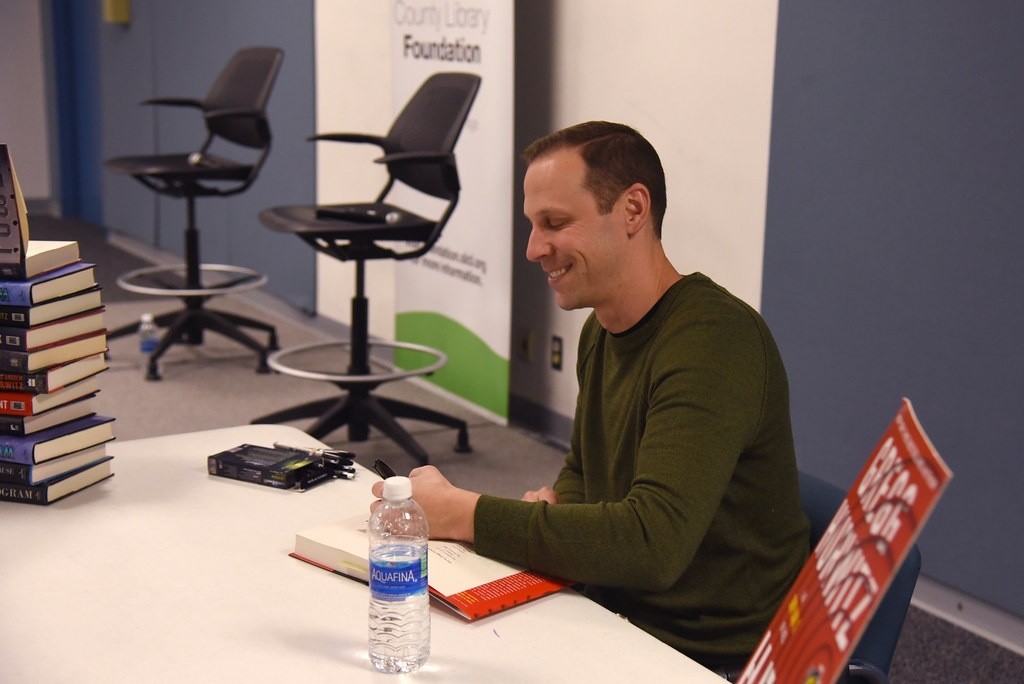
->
[370,120,810,670]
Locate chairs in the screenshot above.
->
[100,44,287,382]
[256,70,481,466]
[797,469,922,684]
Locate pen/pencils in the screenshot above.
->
[372,457,397,479]
[273,442,356,480]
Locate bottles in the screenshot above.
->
[367,476,432,675]
[138,313,162,375]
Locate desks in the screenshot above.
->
[0,426,731,684]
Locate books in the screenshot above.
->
[0,143,116,506]
[289,515,581,620]
